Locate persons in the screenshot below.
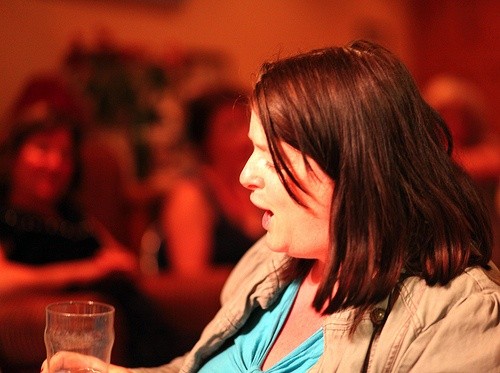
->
[163,87,268,281]
[44,38,500,372]
[2,117,194,368]
[6,32,239,223]
[421,66,500,189]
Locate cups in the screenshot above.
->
[44,301,116,373]
